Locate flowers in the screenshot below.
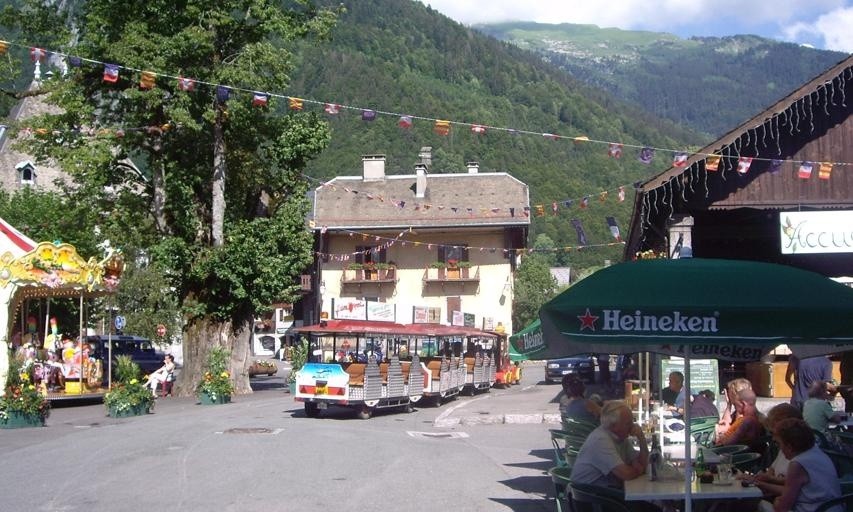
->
[363,262,375,270]
[448,259,458,263]
[0,337,52,426]
[102,353,158,419]
[253,358,275,369]
[284,335,310,383]
[193,346,236,403]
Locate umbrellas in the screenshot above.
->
[509,267,664,457]
[538,258,853,512]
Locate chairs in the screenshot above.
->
[156,371,176,399]
[548,394,853,509]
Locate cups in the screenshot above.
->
[719,453,732,465]
[717,464,732,483]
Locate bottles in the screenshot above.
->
[646,434,661,480]
[695,447,705,477]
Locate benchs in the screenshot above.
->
[324,351,481,388]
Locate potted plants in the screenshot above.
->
[457,261,473,278]
[349,263,364,280]
[432,262,446,280]
[375,262,389,280]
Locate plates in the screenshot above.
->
[713,480,733,484]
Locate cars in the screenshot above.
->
[544,353,595,386]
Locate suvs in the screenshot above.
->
[80,335,175,386]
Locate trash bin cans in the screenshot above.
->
[285,348,292,361]
[624,379,650,410]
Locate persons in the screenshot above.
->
[142,354,175,399]
[649,354,852,511]
[560,354,648,511]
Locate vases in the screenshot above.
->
[450,262,456,267]
[107,398,149,417]
[248,366,278,377]
[1,407,45,428]
[364,269,371,281]
[287,382,296,393]
[198,389,232,405]
